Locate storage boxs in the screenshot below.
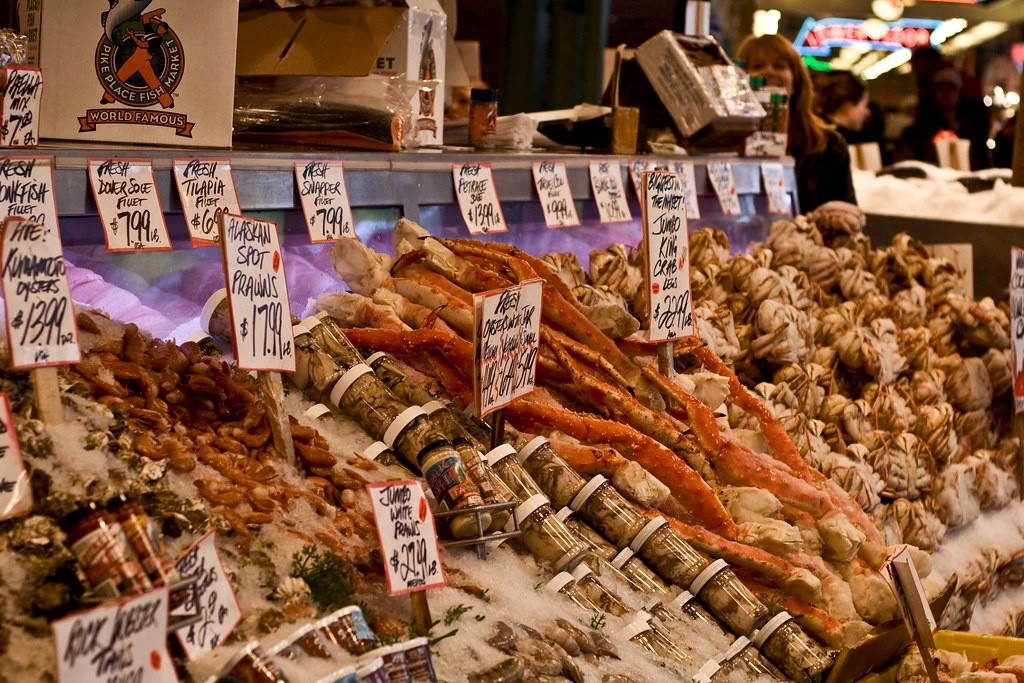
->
[235,0,450,154]
[863,212,1024,399]
[635,28,768,135]
[18,0,239,147]
[609,45,639,153]
[445,37,487,146]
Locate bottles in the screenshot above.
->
[734,59,789,134]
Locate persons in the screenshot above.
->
[739,33,858,217]
[817,44,1022,192]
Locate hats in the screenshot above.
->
[934,69,961,90]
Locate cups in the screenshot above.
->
[15,34,28,64]
[3,27,18,65]
[613,106,640,155]
[0,30,17,65]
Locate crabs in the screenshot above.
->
[282,197,1024,636]
